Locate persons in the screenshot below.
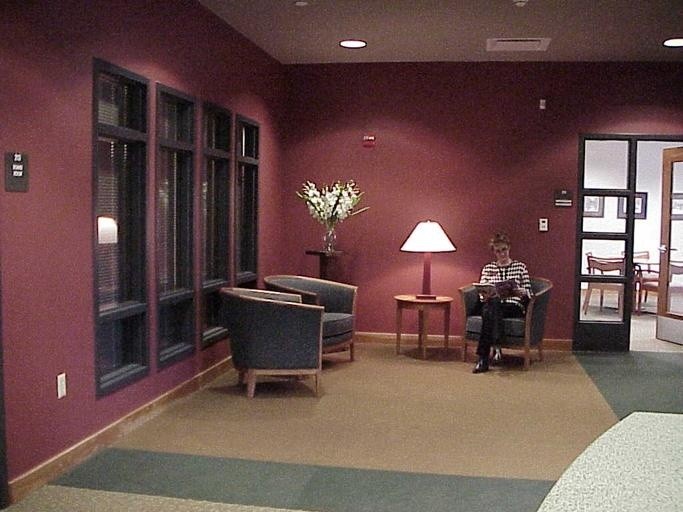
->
[472,233,533,373]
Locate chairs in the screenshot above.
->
[220,287,325,399]
[458,276,554,370]
[262,275,359,361]
[583,249,683,316]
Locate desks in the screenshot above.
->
[393,294,454,360]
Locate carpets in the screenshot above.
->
[537,411,683,512]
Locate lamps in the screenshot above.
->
[400,219,456,299]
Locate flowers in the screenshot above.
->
[295,179,370,231]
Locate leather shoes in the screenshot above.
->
[470,355,489,374]
[490,346,503,362]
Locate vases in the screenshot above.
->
[322,228,336,252]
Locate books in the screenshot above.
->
[472,277,520,300]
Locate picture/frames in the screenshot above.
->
[617,192,648,219]
[670,193,683,221]
[582,196,604,217]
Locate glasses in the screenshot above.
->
[493,244,509,254]
